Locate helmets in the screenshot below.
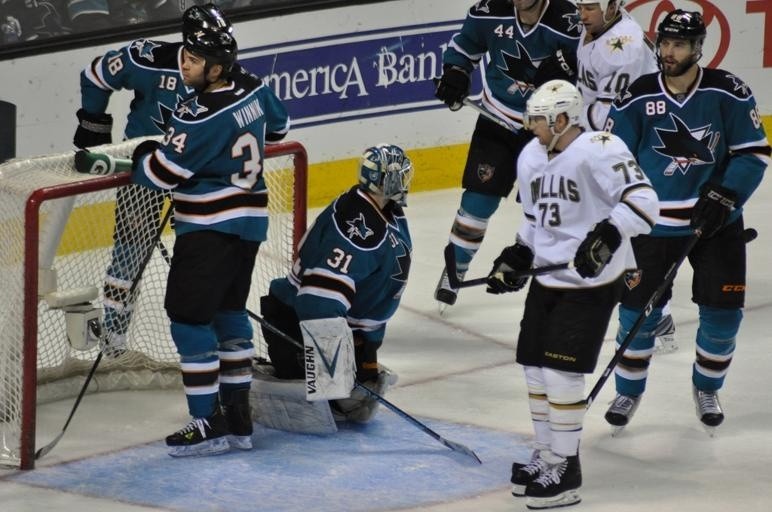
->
[360,143,415,207]
[573,0,624,10]
[182,3,233,34]
[186,28,238,63]
[526,79,583,126]
[658,9,706,40]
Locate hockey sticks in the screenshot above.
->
[445,243,575,289]
[33,200,177,461]
[584,222,707,414]
[247,309,484,466]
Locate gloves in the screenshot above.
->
[74,108,112,149]
[574,218,621,279]
[434,63,470,110]
[486,243,534,294]
[694,181,737,239]
[131,140,160,170]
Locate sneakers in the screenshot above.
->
[166,398,230,446]
[511,443,551,485]
[605,392,641,426]
[656,314,675,336]
[434,265,465,305]
[222,389,252,436]
[525,440,582,498]
[693,383,724,426]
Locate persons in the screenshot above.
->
[433,0,578,313]
[610,10,771,425]
[126,27,289,458]
[487,78,660,509]
[74,4,236,359]
[259,144,413,425]
[576,1,678,354]
